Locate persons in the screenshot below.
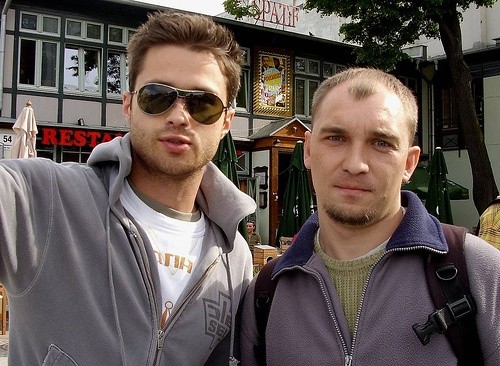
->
[477,193,500,252]
[236,67,500,366]
[0,11,257,366]
[245,220,261,258]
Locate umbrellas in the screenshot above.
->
[275,141,315,248]
[10,99,38,159]
[425,147,454,224]
[211,129,248,241]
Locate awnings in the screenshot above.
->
[401,166,469,200]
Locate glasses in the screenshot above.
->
[129,83,231,125]
